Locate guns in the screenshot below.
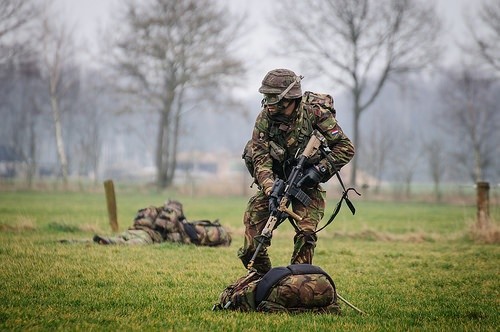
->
[247,129,328,269]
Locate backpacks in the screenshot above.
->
[211,264,338,312]
[301,91,336,119]
[134,206,179,233]
[242,138,292,190]
[183,219,232,247]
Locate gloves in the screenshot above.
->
[269,191,289,218]
[296,165,323,190]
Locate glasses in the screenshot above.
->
[260,94,282,108]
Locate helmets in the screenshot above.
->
[258,68,304,99]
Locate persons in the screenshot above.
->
[93,199,184,245]
[238,68,354,272]
